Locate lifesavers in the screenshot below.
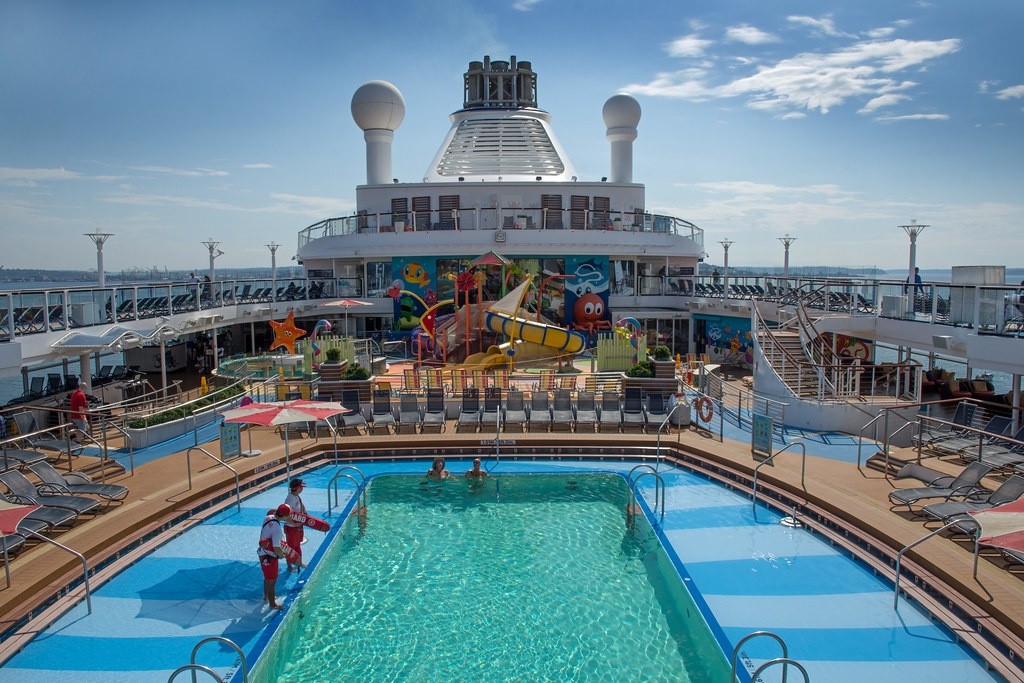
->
[698,395,713,423]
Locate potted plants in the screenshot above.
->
[632,224,639,231]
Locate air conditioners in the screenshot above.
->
[883,295,908,319]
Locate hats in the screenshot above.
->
[277,504,291,516]
[290,479,306,490]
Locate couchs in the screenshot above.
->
[856,361,1024,407]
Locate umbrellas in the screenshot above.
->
[319,298,374,342]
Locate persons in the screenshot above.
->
[70,382,89,444]
[905,267,924,312]
[308,281,319,299]
[284,478,307,572]
[202,276,211,292]
[464,459,489,477]
[427,459,455,479]
[196,343,204,374]
[710,269,720,284]
[257,503,294,609]
[331,320,338,335]
[185,272,201,301]
[659,266,666,280]
[288,282,295,300]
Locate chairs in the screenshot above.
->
[503,216,536,229]
[6,365,141,408]
[712,350,750,377]
[275,369,670,439]
[669,279,883,316]
[888,401,1024,570]
[0,281,325,337]
[0,411,131,587]
[1005,295,1024,330]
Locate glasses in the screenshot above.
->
[473,461,478,464]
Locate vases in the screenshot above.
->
[380,226,413,232]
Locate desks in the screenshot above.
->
[519,218,526,228]
[613,221,622,231]
[394,222,405,232]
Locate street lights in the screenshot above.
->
[777,237,798,303]
[82,227,115,323]
[717,239,736,300]
[897,225,931,318]
[201,238,222,307]
[264,241,282,302]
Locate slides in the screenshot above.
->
[462,309,586,377]
[410,313,455,356]
